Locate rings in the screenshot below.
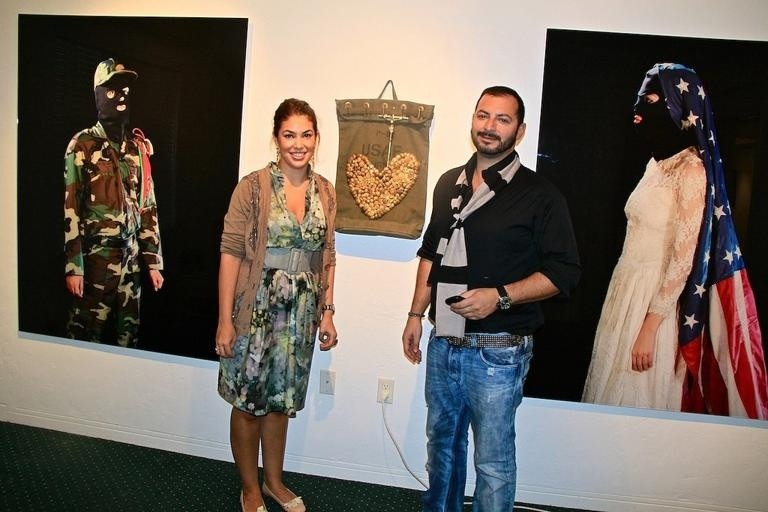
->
[332,339,338,346]
[214,348,220,352]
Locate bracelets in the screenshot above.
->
[407,311,426,318]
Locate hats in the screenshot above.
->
[93,56,138,87]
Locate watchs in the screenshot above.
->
[320,303,335,316]
[495,285,513,311]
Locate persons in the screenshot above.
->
[402,85,582,512]
[216,98,337,512]
[61,56,164,348]
[581,62,707,411]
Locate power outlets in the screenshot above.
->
[377,378,394,404]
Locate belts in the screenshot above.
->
[443,333,532,348]
[90,235,137,248]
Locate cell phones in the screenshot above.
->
[444,295,465,306]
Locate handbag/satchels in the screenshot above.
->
[333,78,438,242]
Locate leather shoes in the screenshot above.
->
[240,487,267,512]
[262,478,307,512]
